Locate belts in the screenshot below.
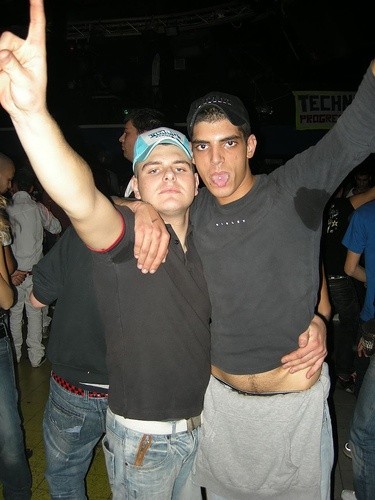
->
[51,370,109,399]
[0,326,9,339]
[106,406,202,435]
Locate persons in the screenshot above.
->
[314,153,375,499]
[117,108,172,200]
[33,225,114,500]
[0,153,62,500]
[0,1,332,500]
[107,57,375,500]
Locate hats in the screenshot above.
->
[132,127,196,171]
[186,91,251,132]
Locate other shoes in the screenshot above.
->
[32,354,47,368]
[341,489,357,500]
[343,442,352,460]
